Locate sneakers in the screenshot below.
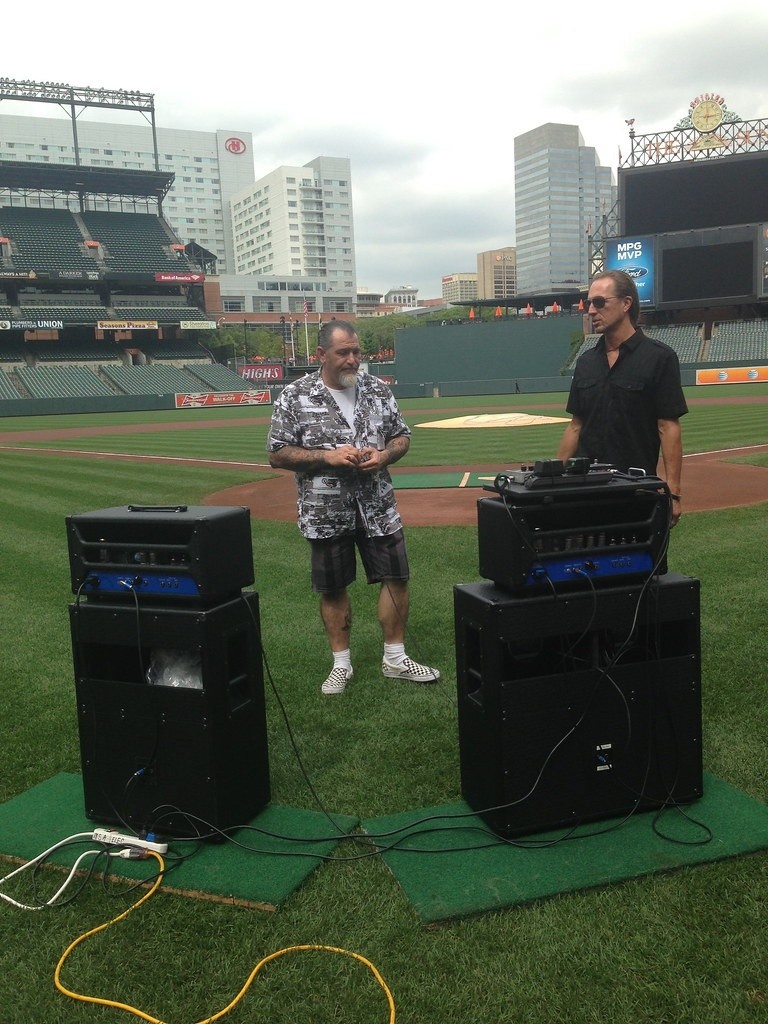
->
[321,666,353,693]
[382,657,440,683]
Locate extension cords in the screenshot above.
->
[90,827,168,854]
[525,471,614,489]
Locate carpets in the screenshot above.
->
[0,771,360,913]
[362,769,767,922]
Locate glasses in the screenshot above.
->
[583,295,617,312]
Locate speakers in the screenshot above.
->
[69,589,273,842]
[454,573,705,840]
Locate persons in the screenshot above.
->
[559,270,689,529]
[266,319,440,694]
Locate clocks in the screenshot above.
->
[692,101,724,132]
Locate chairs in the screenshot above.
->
[0,206,264,400]
[570,323,704,370]
[706,318,768,362]
